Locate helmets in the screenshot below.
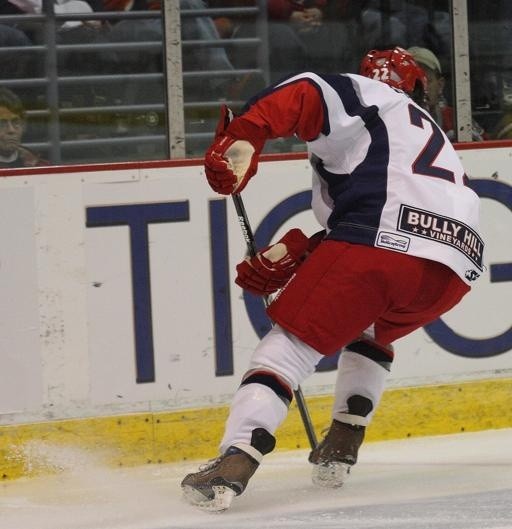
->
[360,46,426,107]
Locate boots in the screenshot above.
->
[181,428,276,500]
[309,395,373,467]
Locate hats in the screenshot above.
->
[406,46,442,74]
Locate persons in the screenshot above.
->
[0,87,50,168]
[181,44,485,498]
[0,1,257,102]
[227,1,512,142]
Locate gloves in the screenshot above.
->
[235,228,326,295]
[204,104,267,195]
